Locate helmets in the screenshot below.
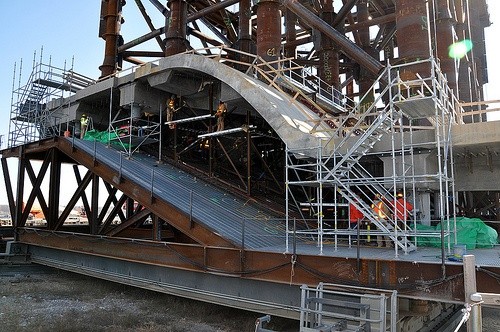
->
[375,194,380,199]
[82,114,85,116]
[396,193,403,198]
[171,95,175,98]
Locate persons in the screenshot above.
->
[216,99,226,131]
[166,95,176,126]
[389,194,412,250]
[350,196,363,243]
[80,114,88,139]
[371,193,391,247]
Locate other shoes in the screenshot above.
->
[353,240,357,242]
[386,244,394,247]
[378,244,384,247]
